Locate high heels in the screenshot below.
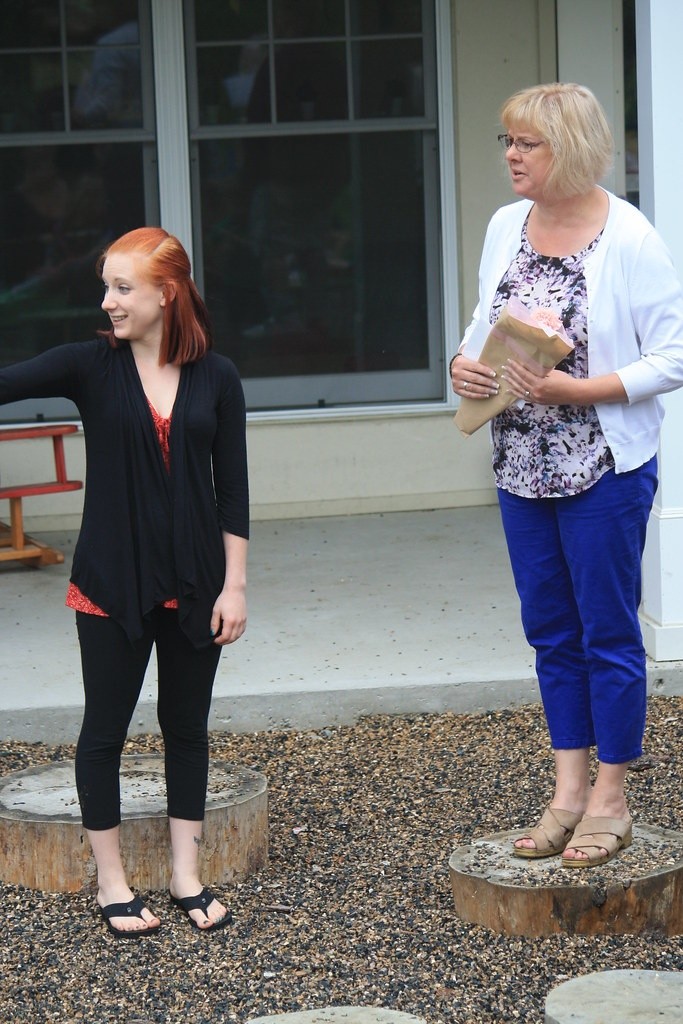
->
[561,814,632,867]
[513,807,583,857]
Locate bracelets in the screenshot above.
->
[449,353,462,375]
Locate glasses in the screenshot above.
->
[498,134,547,153]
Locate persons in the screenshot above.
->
[447,82,683,866]
[0,227,248,937]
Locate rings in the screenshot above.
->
[464,382,467,389]
[525,391,529,397]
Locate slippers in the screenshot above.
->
[99,894,159,939]
[169,886,231,931]
